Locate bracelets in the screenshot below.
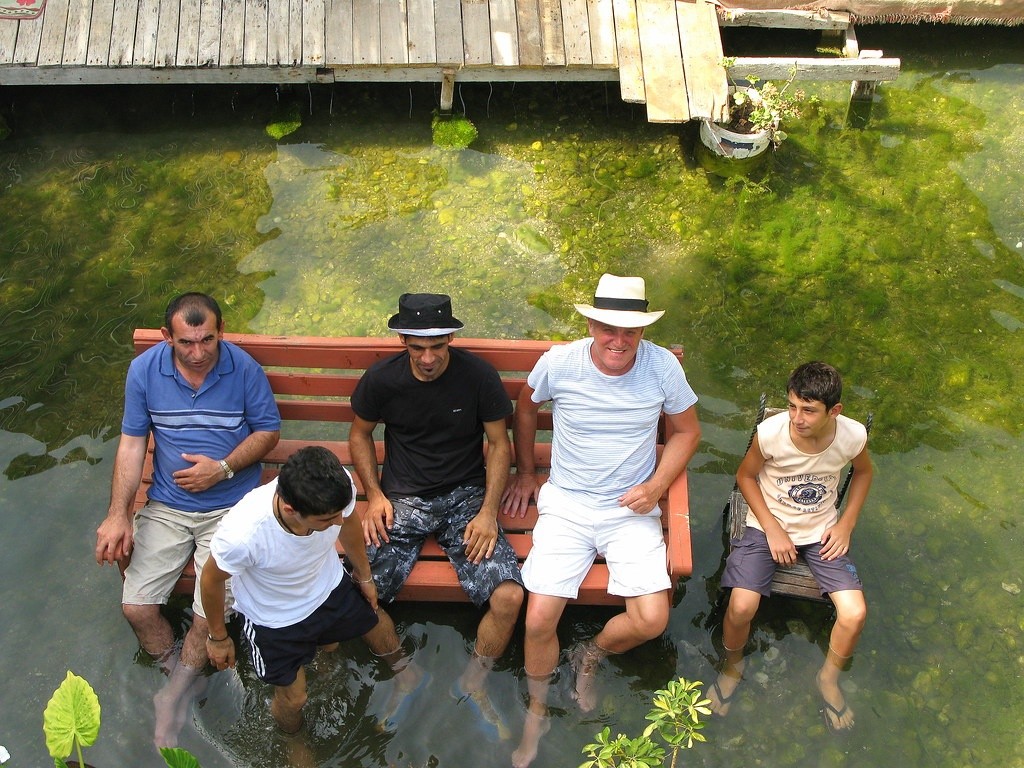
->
[206,633,229,642]
[350,572,374,584]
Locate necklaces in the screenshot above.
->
[277,494,310,536]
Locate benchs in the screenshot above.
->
[101,329,693,608]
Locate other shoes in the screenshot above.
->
[374,670,430,736]
[449,678,513,744]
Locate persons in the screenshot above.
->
[704,361,873,735]
[95,292,281,759]
[348,293,526,744]
[499,273,702,768]
[201,445,380,768]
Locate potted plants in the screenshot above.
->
[695,56,827,178]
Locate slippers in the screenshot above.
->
[811,665,856,737]
[704,658,740,721]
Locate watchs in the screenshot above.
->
[219,460,234,480]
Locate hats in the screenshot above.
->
[574,273,665,328]
[387,292,465,336]
